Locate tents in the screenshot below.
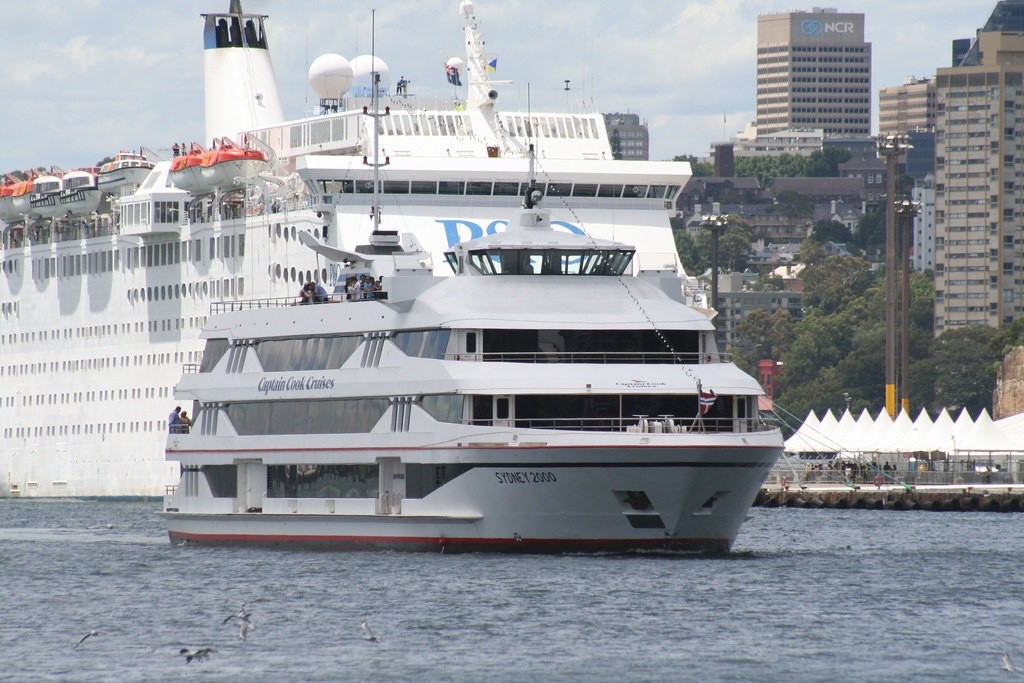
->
[782,406,1024,471]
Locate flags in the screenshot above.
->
[488,59,496,71]
[444,63,455,75]
[699,392,717,414]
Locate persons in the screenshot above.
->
[805,460,899,484]
[299,282,328,304]
[172,143,187,157]
[168,406,192,434]
[344,275,387,301]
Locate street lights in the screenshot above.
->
[893,199,923,418]
[876,133,914,422]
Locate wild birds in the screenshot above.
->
[360,617,380,645]
[178,648,217,665]
[72,630,115,647]
[87,523,129,531]
[221,608,255,640]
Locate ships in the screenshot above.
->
[0,1,692,503]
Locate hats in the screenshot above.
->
[303,282,310,288]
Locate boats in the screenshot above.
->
[0,136,269,224]
[163,72,785,555]
